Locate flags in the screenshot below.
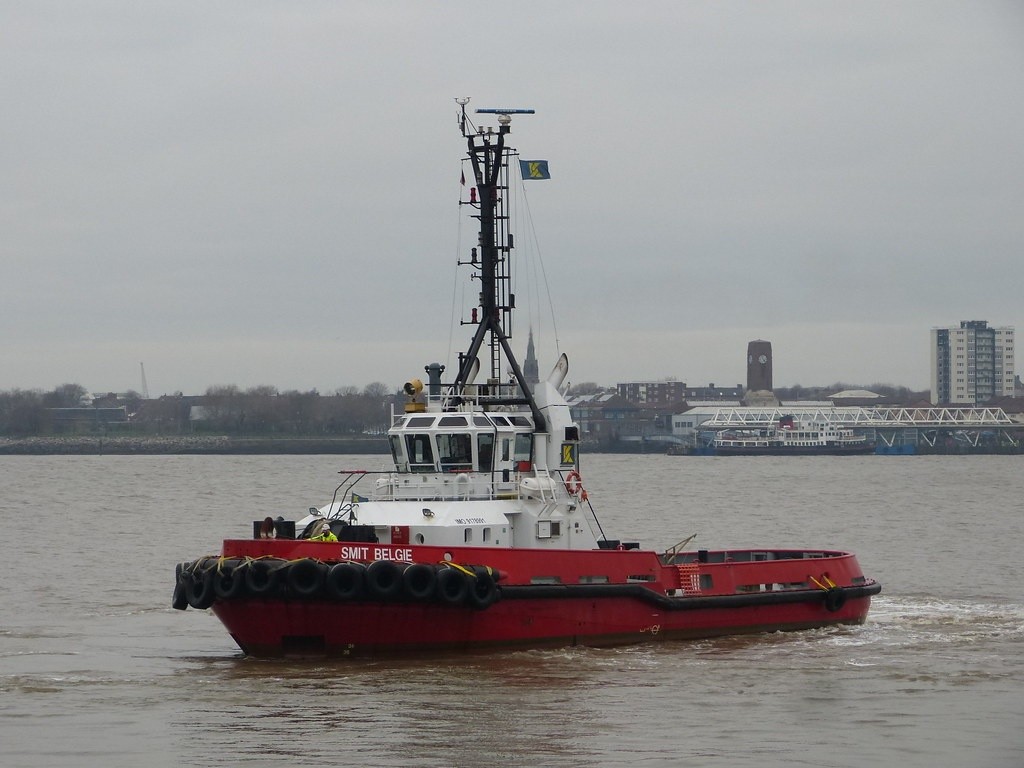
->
[352,493,368,503]
[519,160,551,180]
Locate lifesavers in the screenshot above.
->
[826,588,845,612]
[173,561,492,611]
[566,471,582,494]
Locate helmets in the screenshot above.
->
[322,524,330,531]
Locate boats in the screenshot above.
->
[666,415,876,455]
[172,94,884,658]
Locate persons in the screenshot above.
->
[304,524,338,542]
[259,517,277,539]
